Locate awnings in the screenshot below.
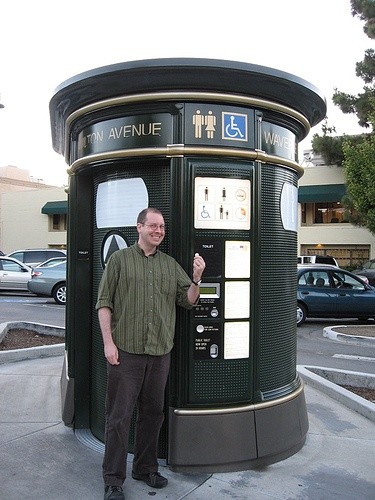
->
[41,201,68,214]
[298,184,347,203]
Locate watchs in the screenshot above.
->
[192,279,202,286]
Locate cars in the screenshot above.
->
[296,264,375,326]
[0,256,31,293]
[26,257,68,304]
[297,255,338,268]
[6,249,67,267]
[350,260,375,284]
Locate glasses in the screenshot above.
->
[140,222,166,229]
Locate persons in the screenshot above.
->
[95,208,205,500]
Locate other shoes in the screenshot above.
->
[104,469,169,500]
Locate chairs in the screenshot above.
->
[331,218,339,223]
[306,277,314,287]
[316,278,325,287]
